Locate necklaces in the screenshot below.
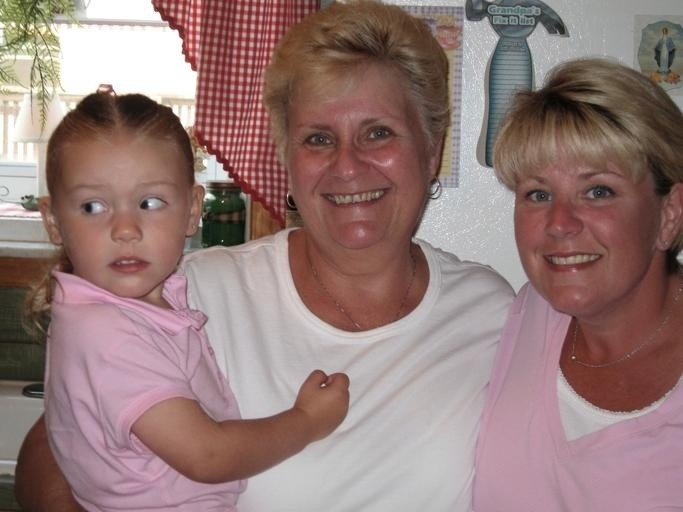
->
[569,272,683,369]
[299,224,418,334]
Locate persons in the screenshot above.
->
[11,0,517,512]
[20,83,352,512]
[470,55,682,512]
[652,26,675,74]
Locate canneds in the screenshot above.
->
[202,180,246,248]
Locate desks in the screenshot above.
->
[0,241,62,381]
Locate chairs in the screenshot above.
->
[0,458,24,512]
